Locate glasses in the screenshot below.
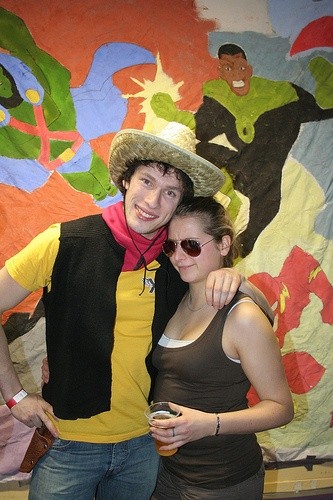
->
[162,238,215,257]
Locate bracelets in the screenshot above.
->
[6,387,28,410]
[213,412,221,437]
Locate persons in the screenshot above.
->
[145,197,295,500]
[0,120,248,500]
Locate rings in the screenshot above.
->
[172,427,175,436]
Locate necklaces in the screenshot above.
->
[187,291,211,313]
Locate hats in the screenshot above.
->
[109,122,226,197]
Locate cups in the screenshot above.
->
[144,402,181,456]
